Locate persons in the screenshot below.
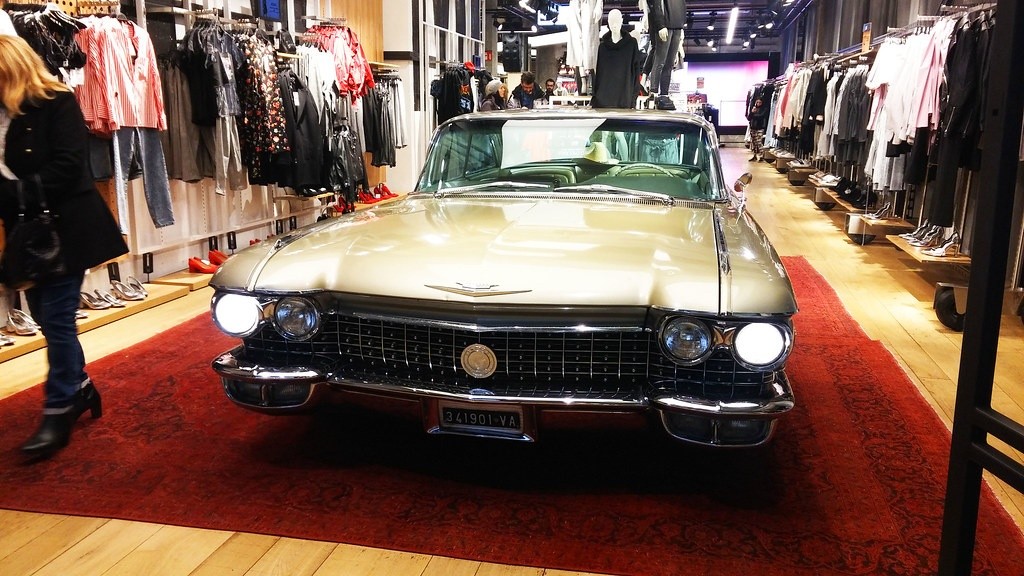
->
[0,35,130,461]
[480,71,569,111]
[747,97,766,162]
[566,0,686,109]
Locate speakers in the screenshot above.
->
[502,33,522,72]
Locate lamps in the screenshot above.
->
[493,0,559,44]
[687,0,794,51]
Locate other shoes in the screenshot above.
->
[74,308,88,318]
[769,147,842,187]
[749,156,757,162]
[0,332,13,346]
[250,239,261,245]
[758,157,764,163]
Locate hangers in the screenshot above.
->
[235,17,268,43]
[297,32,328,52]
[885,2,994,44]
[2,0,87,32]
[194,8,226,33]
[333,117,350,138]
[78,1,131,26]
[857,55,869,75]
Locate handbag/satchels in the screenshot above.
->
[4,175,67,291]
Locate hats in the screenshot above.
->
[485,80,505,96]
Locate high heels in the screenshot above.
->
[22,413,72,456]
[327,184,399,214]
[6,308,41,335]
[126,275,148,297]
[209,249,229,265]
[44,379,101,423]
[78,291,111,309]
[862,202,893,219]
[909,225,943,246]
[95,289,124,307]
[189,256,218,273]
[828,177,877,209]
[110,280,144,301]
[921,232,960,258]
[898,219,931,240]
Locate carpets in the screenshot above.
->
[1,256,1024,576]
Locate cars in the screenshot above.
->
[208,107,798,450]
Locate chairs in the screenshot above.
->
[607,166,691,180]
[498,166,577,184]
